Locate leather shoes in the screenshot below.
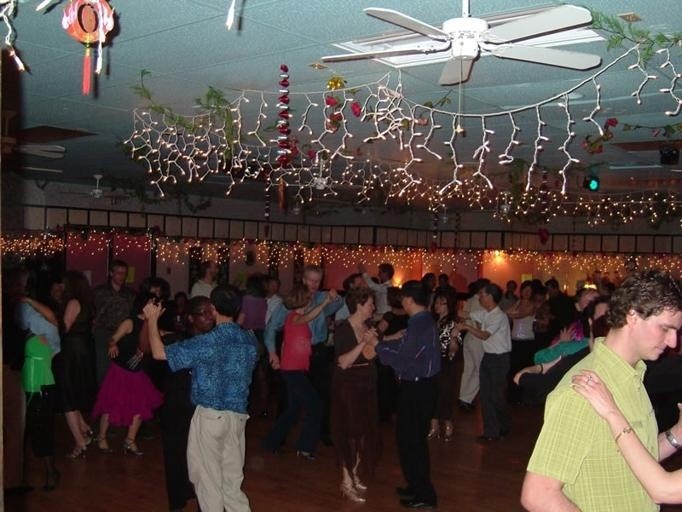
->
[466,424,512,443]
[456,401,478,410]
[319,434,334,446]
[394,486,437,509]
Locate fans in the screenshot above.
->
[321,0,604,89]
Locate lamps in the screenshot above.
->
[582,174,602,193]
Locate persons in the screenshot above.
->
[520,274,681,511]
[1,258,58,493]
[330,287,407,502]
[364,281,441,510]
[138,284,250,511]
[41,260,534,460]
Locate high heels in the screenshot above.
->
[39,470,62,491]
[338,478,368,504]
[425,427,457,444]
[62,435,144,463]
[256,443,317,462]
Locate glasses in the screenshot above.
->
[189,309,213,317]
[434,301,448,305]
